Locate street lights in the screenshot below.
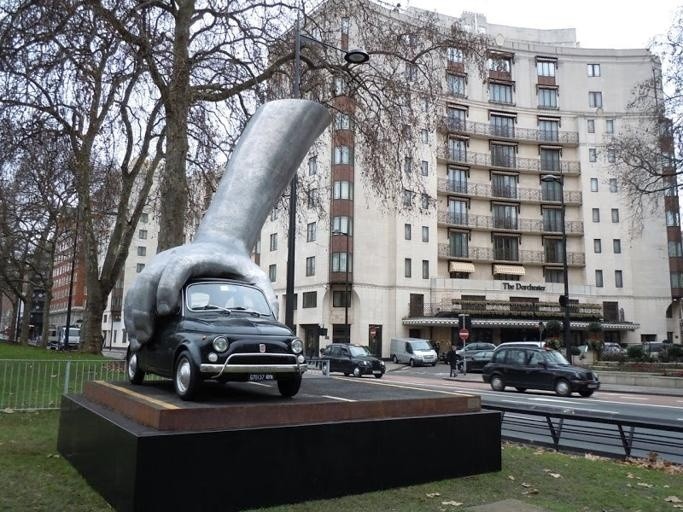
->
[539,172,572,365]
[282,16,368,331]
[62,202,118,351]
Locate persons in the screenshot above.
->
[437,343,440,355]
[448,346,457,377]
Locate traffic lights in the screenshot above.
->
[558,293,566,308]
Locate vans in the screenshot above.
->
[389,336,438,367]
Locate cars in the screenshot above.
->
[494,340,683,363]
[3,320,53,351]
[457,350,494,373]
[123,276,308,401]
[454,343,497,360]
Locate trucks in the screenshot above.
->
[46,323,80,350]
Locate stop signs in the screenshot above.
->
[458,329,470,340]
[370,329,376,336]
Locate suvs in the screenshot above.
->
[319,342,386,379]
[481,344,601,399]
